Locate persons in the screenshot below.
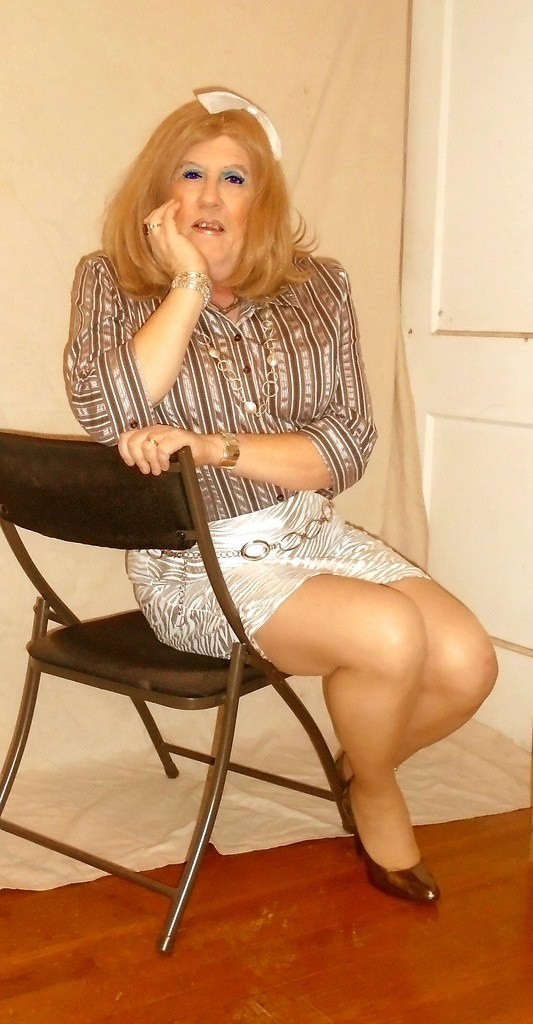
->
[65,86,500,901]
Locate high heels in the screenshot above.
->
[342,773,440,903]
[330,749,351,790]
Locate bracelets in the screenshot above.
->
[170,272,214,308]
[212,431,240,470]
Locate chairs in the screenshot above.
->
[0,426,355,953]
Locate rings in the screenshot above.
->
[143,222,160,235]
[146,437,158,447]
